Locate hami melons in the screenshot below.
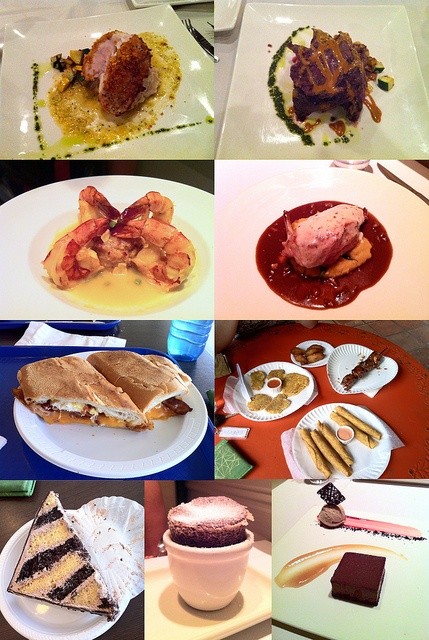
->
[316,482,346,527]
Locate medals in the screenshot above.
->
[87,351,193,420]
[13,356,154,432]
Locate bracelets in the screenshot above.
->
[336,425,354,444]
[266,377,282,393]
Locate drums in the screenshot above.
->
[376,161,428,206]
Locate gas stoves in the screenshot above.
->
[7,491,120,622]
[167,496,254,548]
[330,553,387,607]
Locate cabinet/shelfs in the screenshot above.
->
[165,320,213,362]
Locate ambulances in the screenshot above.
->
[335,406,382,440]
[309,431,353,477]
[330,411,379,449]
[300,428,332,478]
[316,420,354,467]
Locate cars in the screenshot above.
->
[162,528,256,612]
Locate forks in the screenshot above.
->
[181,18,214,57]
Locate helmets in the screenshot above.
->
[326,343,398,394]
[233,360,315,422]
[0,175,214,320]
[144,544,272,640]
[12,349,208,478]
[0,3,213,161]
[292,403,391,479]
[291,340,335,368]
[214,3,428,159]
[214,0,243,31]
[214,167,429,320]
[1,509,131,640]
[69,496,144,599]
[272,479,428,639]
